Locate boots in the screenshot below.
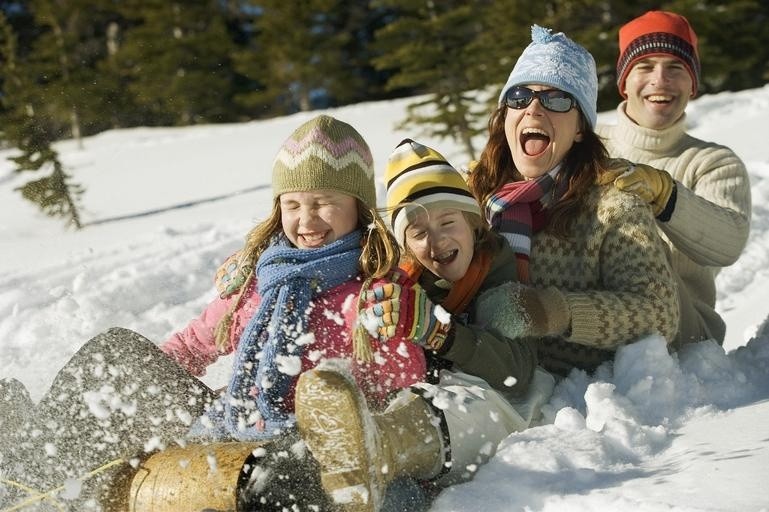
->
[295,360,446,512]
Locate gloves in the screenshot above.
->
[595,158,674,218]
[473,282,571,342]
[359,270,451,354]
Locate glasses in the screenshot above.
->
[505,86,583,113]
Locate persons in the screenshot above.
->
[593,11,752,355]
[294,24,682,512]
[158,114,428,441]
[362,137,556,403]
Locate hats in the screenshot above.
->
[616,10,699,100]
[498,23,598,133]
[270,115,377,210]
[384,138,482,252]
